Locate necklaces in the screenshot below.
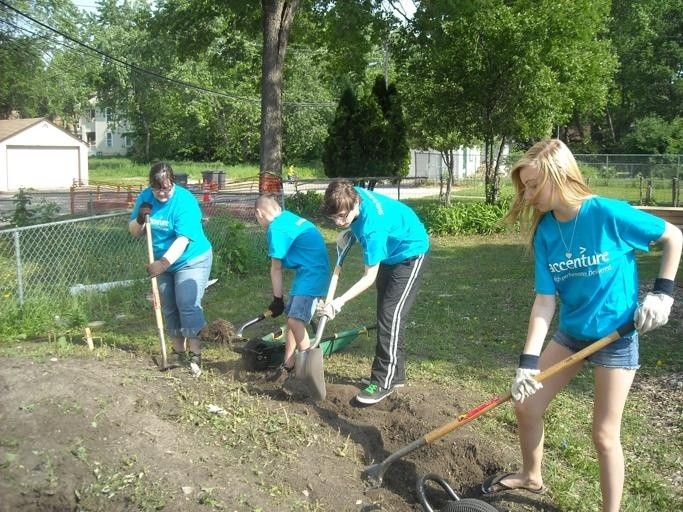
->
[553,205,582,259]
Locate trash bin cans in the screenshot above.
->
[202,170,226,191]
[173,172,188,187]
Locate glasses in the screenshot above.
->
[152,185,172,192]
[328,209,350,219]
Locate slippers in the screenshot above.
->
[482,473,543,494]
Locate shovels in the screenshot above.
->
[360,320,635,488]
[196,308,273,344]
[294,233,356,402]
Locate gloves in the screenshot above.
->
[145,257,170,278]
[634,277,674,334]
[137,203,152,225]
[337,228,352,250]
[511,355,543,404]
[269,295,285,317]
[316,297,344,321]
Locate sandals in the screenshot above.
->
[169,348,200,374]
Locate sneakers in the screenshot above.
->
[278,362,296,374]
[355,377,405,403]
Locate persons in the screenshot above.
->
[315,175,432,405]
[481,138,683,512]
[253,193,332,380]
[127,162,213,374]
